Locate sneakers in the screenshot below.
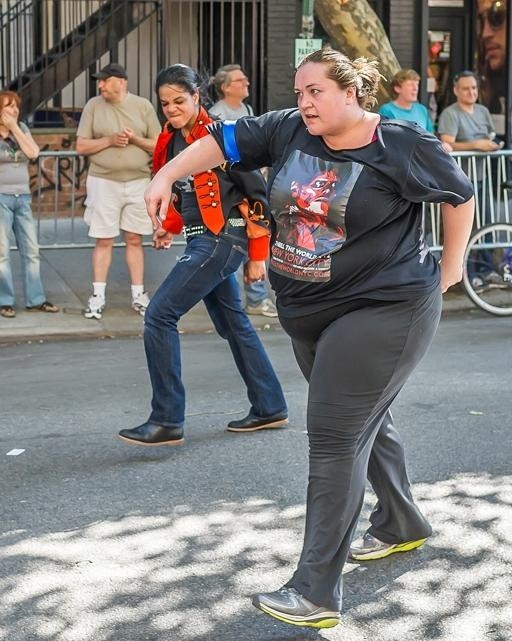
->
[253,586,342,630]
[84,294,106,320]
[459,272,510,296]
[226,412,289,432]
[347,531,429,562]
[24,302,60,313]
[130,292,151,317]
[1,306,16,318]
[244,298,279,318]
[119,423,185,447]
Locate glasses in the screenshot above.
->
[473,2,506,37]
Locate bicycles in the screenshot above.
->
[460,221,512,315]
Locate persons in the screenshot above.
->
[437,70,510,295]
[473,0,507,79]
[378,68,434,137]
[74,62,163,321]
[145,48,474,629]
[0,87,63,318]
[119,63,290,447]
[205,62,279,319]
